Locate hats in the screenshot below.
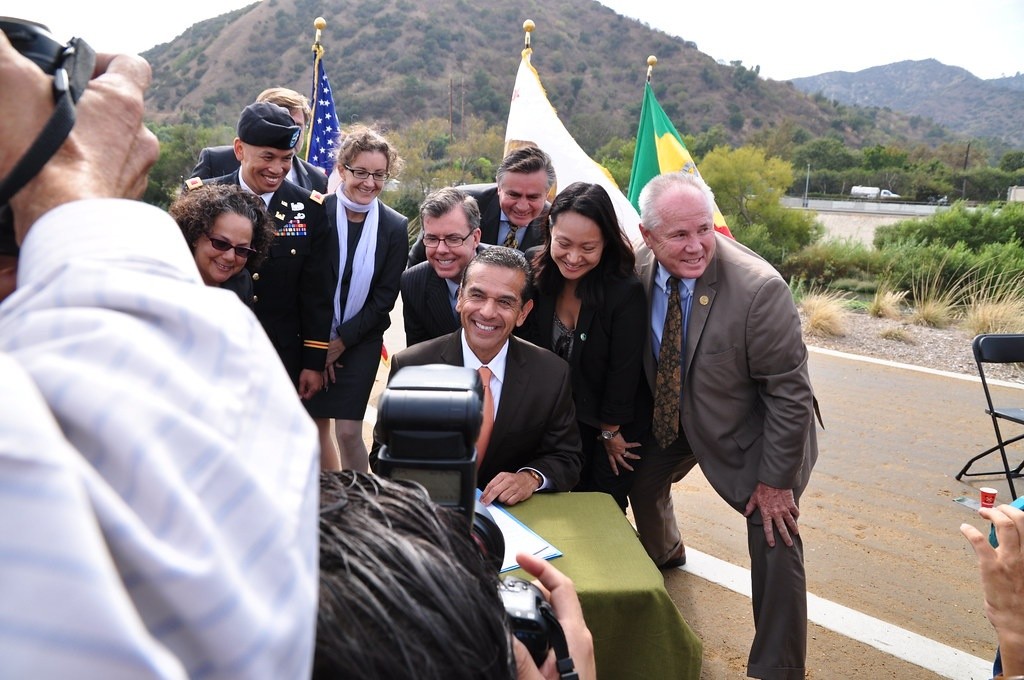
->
[237,102,301,150]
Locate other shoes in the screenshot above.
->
[657,544,686,569]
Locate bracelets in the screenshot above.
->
[995,672,1024,680]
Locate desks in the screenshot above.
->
[500,492,702,679]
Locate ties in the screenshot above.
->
[501,221,519,249]
[652,277,683,449]
[475,368,494,470]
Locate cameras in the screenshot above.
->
[1,15,96,260]
[367,362,556,671]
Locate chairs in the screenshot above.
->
[957,333,1024,503]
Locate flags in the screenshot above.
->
[305,45,340,177]
[628,80,734,241]
[502,47,642,243]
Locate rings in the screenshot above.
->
[623,451,627,457]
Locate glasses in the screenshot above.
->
[422,229,473,248]
[344,164,390,181]
[203,231,256,258]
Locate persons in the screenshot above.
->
[183,87,329,194]
[408,146,555,268]
[0,33,321,680]
[180,102,335,405]
[315,128,408,472]
[399,187,487,348]
[315,470,597,680]
[170,184,273,314]
[628,173,825,680]
[511,182,648,517]
[387,247,582,508]
[959,494,1024,680]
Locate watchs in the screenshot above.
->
[601,429,620,440]
[523,469,542,488]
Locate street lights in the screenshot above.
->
[803,164,810,200]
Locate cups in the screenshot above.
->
[980,487,998,508]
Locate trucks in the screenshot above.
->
[850,184,901,200]
